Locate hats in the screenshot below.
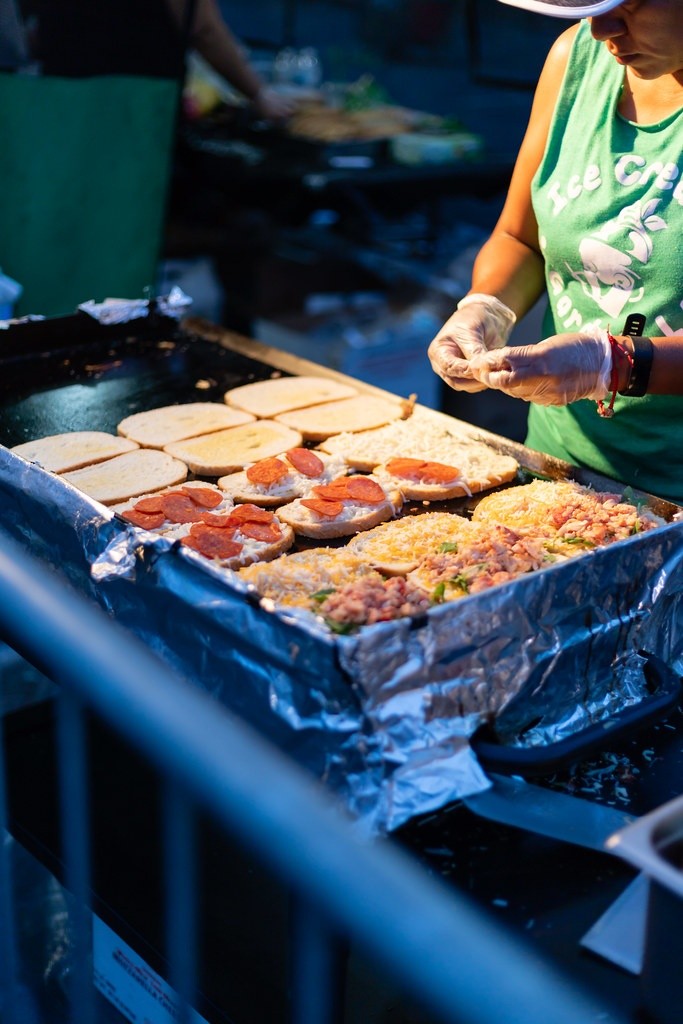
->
[495,0,624,20]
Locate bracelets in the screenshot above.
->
[597,323,632,419]
[618,313,653,397]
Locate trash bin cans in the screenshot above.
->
[0,74,181,319]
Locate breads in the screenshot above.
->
[107,480,234,536]
[224,375,361,418]
[348,512,478,576]
[408,545,570,599]
[165,419,303,475]
[181,514,294,568]
[276,472,405,539]
[60,450,187,505]
[11,431,138,474]
[320,414,450,470]
[216,449,348,506]
[117,404,257,448]
[371,447,518,500]
[236,548,380,608]
[276,394,404,439]
[470,483,597,530]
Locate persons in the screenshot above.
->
[1,0,299,318]
[427,0,683,509]
